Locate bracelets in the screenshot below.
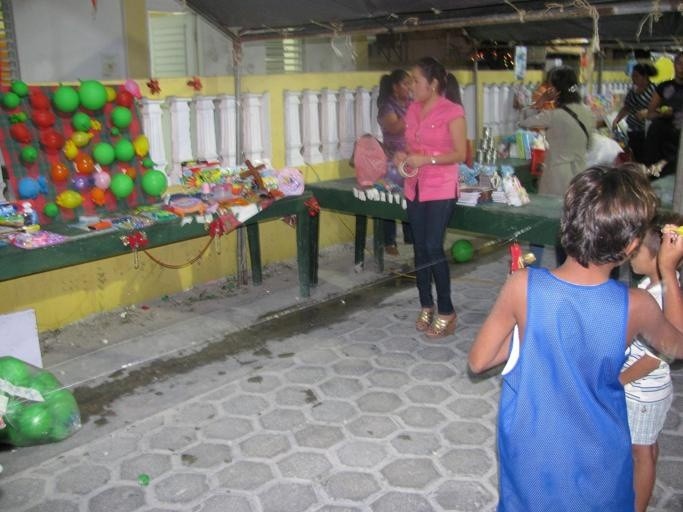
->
[431,155,437,166]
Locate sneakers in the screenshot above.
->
[385,243,399,257]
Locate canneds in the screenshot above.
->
[476,127,497,164]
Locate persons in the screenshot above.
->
[376,69,415,258]
[391,56,468,338]
[516,66,596,269]
[468,162,683,511]
[531,49,681,191]
[616,208,682,511]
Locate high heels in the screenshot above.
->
[415,306,435,333]
[427,314,459,338]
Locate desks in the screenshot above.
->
[629,174,677,288]
[304,176,620,289]
[469,157,543,194]
[0,191,315,298]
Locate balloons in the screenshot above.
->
[2,77,168,216]
[449,238,475,264]
[2,357,78,446]
